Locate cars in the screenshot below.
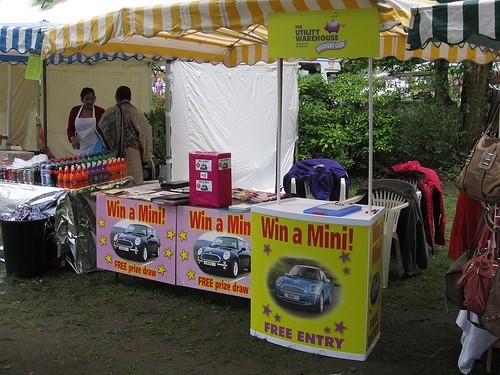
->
[274,264,331,312]
[112,223,160,261]
[197,235,251,277]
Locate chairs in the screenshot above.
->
[283,159,349,202]
[342,160,446,290]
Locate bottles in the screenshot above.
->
[33,149,127,189]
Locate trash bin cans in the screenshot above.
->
[0,217,57,278]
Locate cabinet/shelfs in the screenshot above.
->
[250,197,385,362]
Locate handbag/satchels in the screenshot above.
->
[454,103,500,203]
[445,208,500,337]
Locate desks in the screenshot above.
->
[91,183,274,299]
[0,176,137,275]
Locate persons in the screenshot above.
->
[67,88,105,156]
[98,86,152,182]
